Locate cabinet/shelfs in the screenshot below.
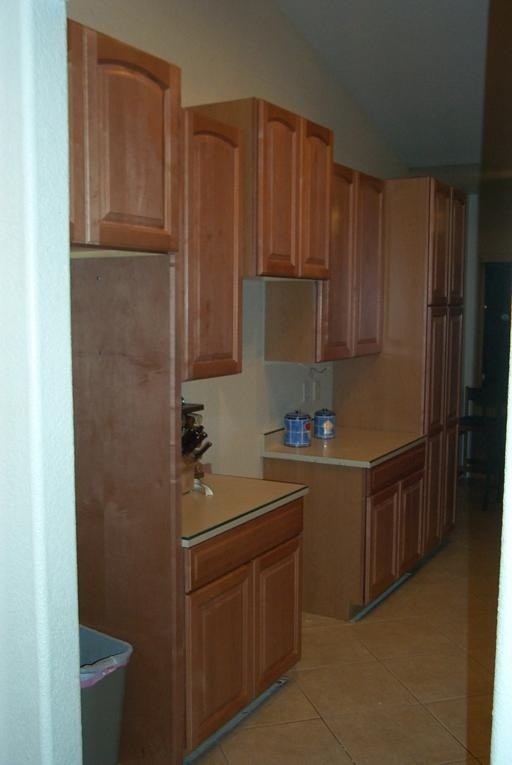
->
[183,494,302,755]
[262,443,427,625]
[332,178,463,551]
[188,98,334,282]
[68,17,180,254]
[267,165,388,365]
[182,109,244,380]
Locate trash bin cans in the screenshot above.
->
[80,624,133,765]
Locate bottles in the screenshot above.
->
[283,408,336,447]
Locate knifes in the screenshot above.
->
[181,423,213,462]
